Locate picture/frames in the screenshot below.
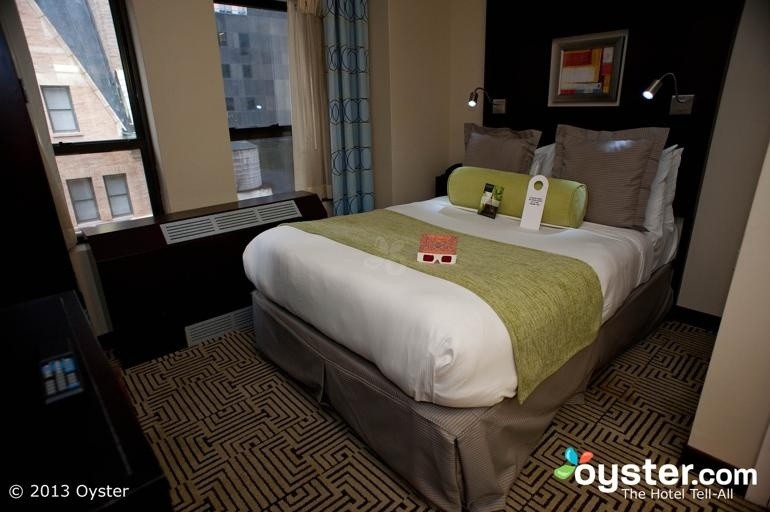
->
[547,30,629,107]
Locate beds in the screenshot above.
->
[242,193,685,512]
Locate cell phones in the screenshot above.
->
[35,337,86,406]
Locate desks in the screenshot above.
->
[0,289,172,511]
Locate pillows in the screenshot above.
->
[447,122,685,238]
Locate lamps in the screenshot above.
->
[467,86,505,114]
[642,72,695,115]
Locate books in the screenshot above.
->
[477,182,505,219]
